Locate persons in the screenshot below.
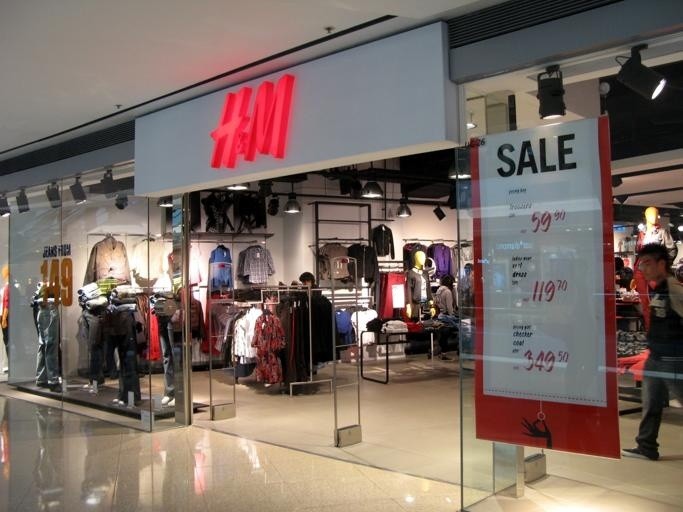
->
[636,207,678,264]
[0,263,9,372]
[299,272,321,295]
[405,251,436,322]
[618,242,683,460]
[435,275,456,361]
[37,295,175,407]
[615,257,633,331]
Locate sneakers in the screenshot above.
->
[622,448,648,459]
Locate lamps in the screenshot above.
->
[226,179,304,218]
[0,169,120,221]
[114,194,176,213]
[336,161,448,223]
[612,43,667,101]
[537,62,567,118]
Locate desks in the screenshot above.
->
[359,327,458,385]
